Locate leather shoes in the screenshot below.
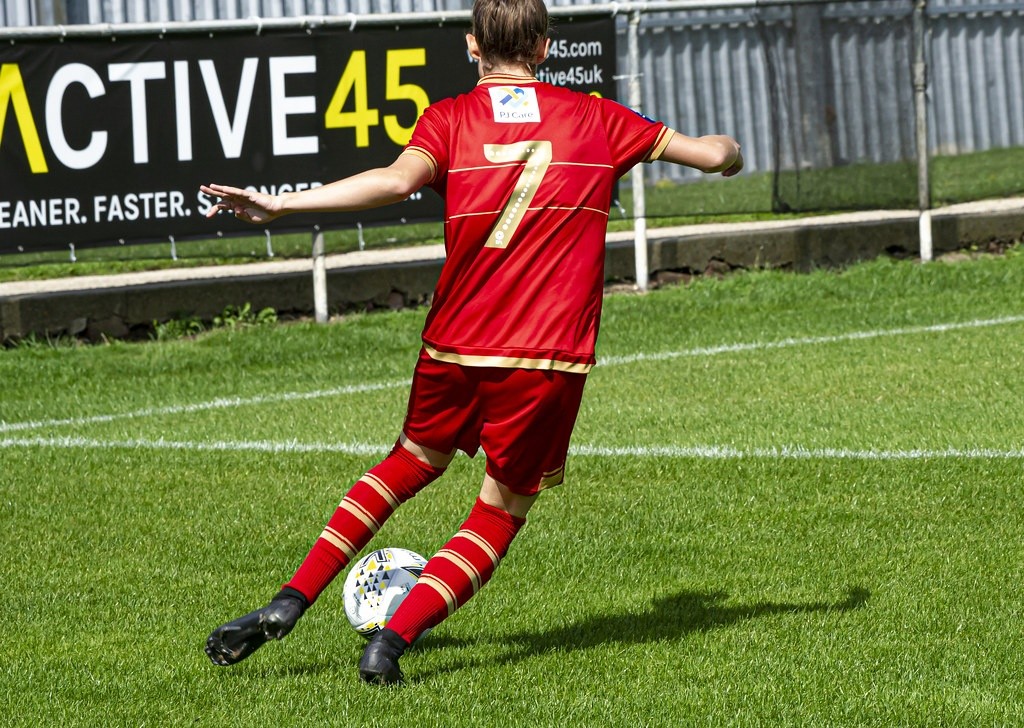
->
[206,599,407,685]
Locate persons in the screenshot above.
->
[205,0,745,684]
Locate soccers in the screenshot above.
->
[341,544,442,642]
